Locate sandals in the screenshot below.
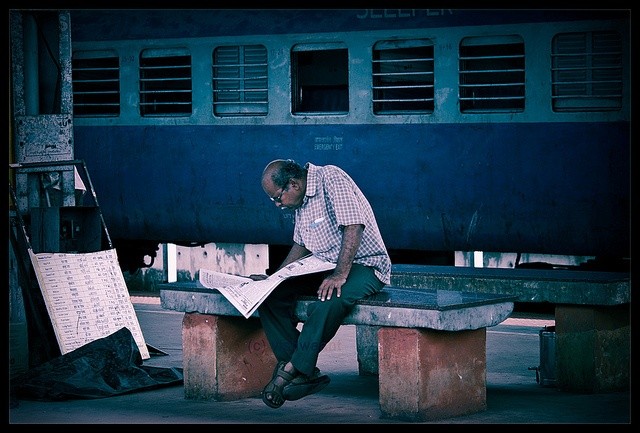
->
[281,366,331,401]
[262,360,296,409]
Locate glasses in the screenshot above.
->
[269,174,300,203]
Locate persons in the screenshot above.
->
[249,158,391,408]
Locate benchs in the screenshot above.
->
[159,279,520,421]
[352,263,637,397]
